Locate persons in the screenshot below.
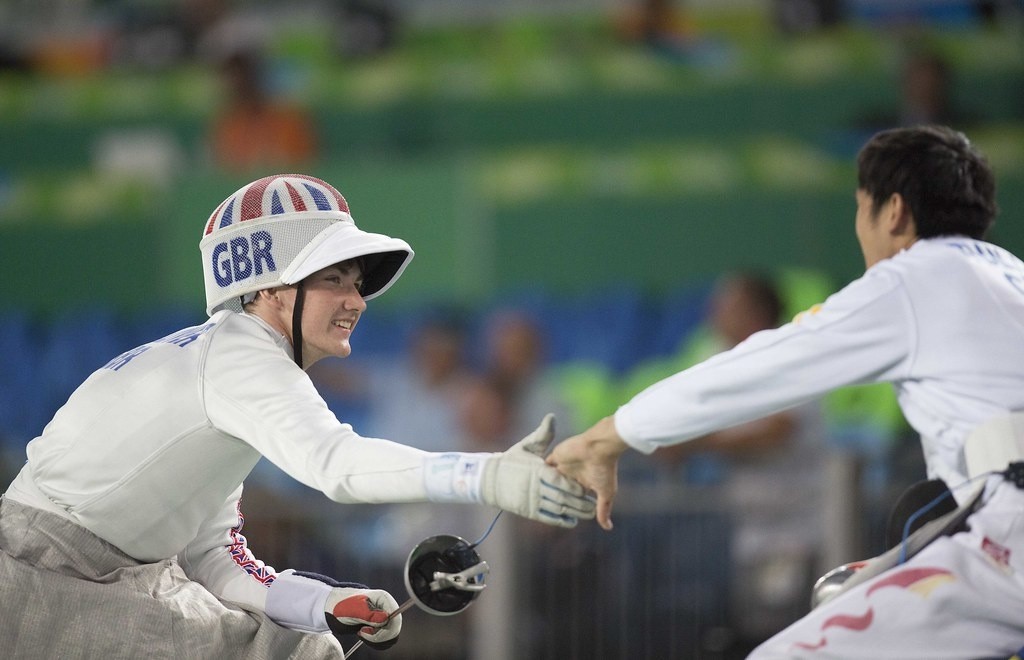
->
[544,122,1024,660]
[0,170,599,659]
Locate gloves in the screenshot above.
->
[265,567,402,650]
[422,413,597,528]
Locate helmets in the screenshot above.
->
[199,173,415,317]
